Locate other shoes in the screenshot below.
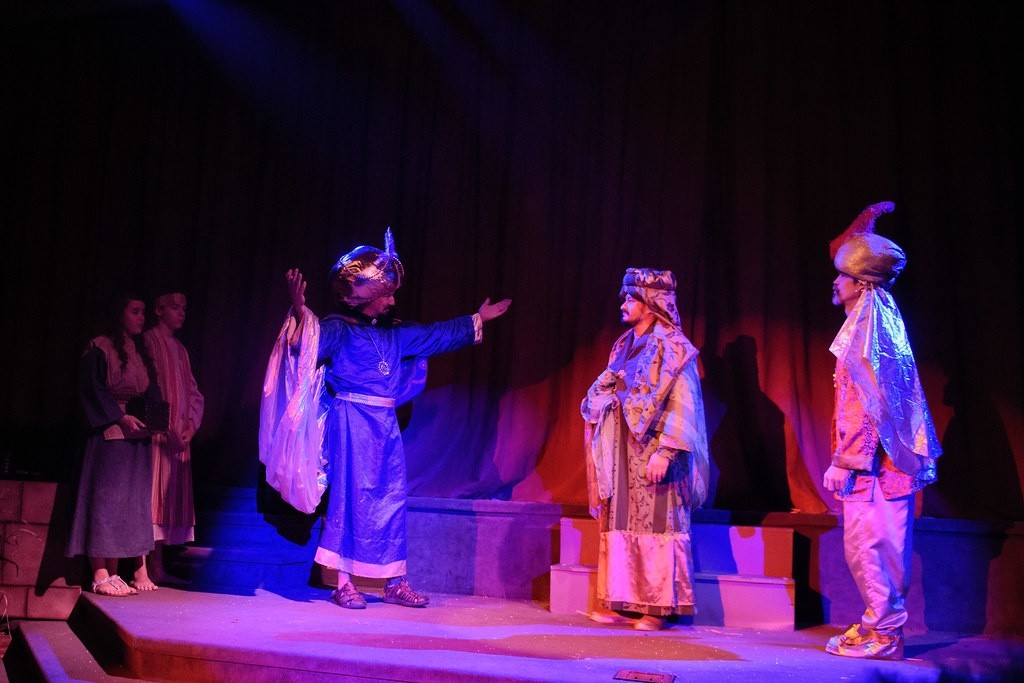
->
[826,625,904,663]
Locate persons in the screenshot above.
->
[820,234,946,663]
[578,267,711,632]
[142,291,205,591]
[74,294,156,598]
[259,224,514,608]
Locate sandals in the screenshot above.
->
[90,573,139,597]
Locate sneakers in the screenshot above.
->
[590,610,640,625]
[381,581,430,606]
[329,581,368,609]
[633,614,668,630]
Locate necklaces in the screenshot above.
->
[364,325,391,376]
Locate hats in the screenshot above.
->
[828,203,907,291]
[620,266,683,333]
[328,225,406,313]
